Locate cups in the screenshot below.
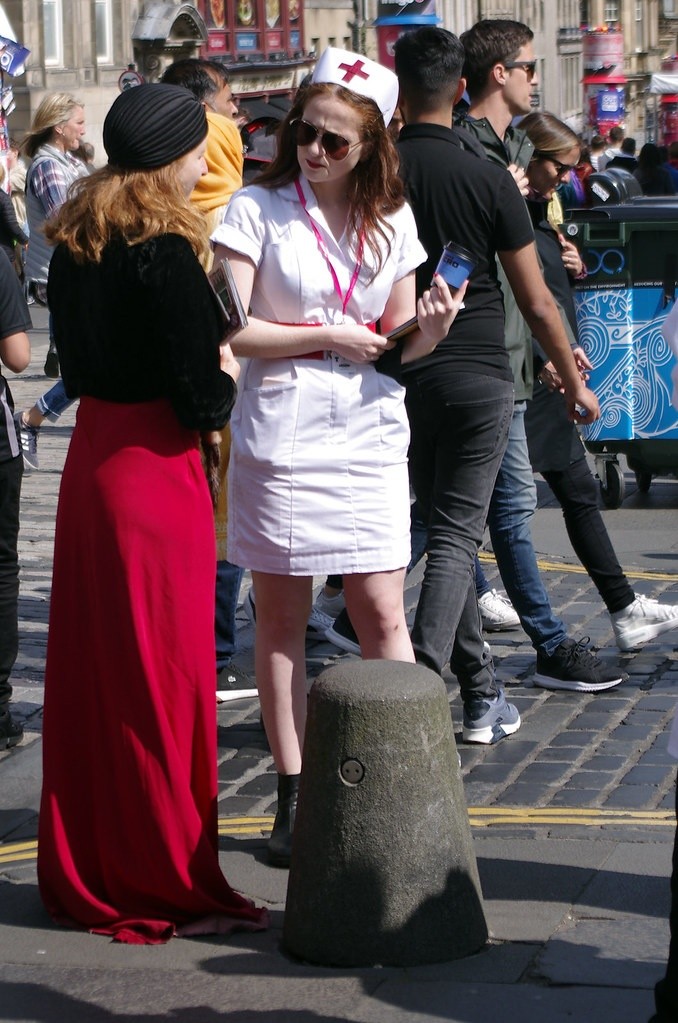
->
[429,241,480,298]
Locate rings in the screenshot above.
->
[371,355,374,362]
[568,257,571,263]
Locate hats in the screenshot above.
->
[104,82,210,174]
[311,46,399,130]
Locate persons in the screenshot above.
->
[375,27,599,767]
[0,91,96,467]
[159,17,678,702]
[0,66,96,307]
[209,46,468,866]
[36,82,267,945]
[0,244,33,747]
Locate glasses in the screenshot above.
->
[504,59,536,83]
[531,150,584,177]
[289,116,366,160]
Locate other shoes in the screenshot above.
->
[43,350,60,379]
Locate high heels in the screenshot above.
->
[267,772,301,868]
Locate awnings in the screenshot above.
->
[651,74,678,95]
[268,96,293,112]
[237,100,289,124]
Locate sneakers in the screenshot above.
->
[460,689,522,744]
[607,593,678,653]
[324,607,362,656]
[242,587,256,629]
[533,636,621,692]
[215,661,259,702]
[13,411,40,471]
[307,587,347,631]
[0,710,24,750]
[477,588,521,630]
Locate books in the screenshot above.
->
[208,258,249,346]
[382,302,465,339]
